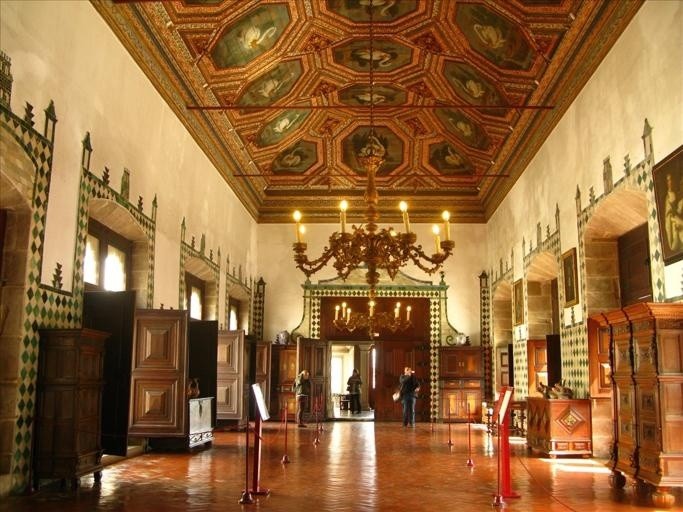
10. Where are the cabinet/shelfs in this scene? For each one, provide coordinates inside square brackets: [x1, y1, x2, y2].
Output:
[602, 300, 681, 498]
[520, 396, 593, 458]
[440, 347, 484, 423]
[149, 392, 214, 451]
[268, 344, 302, 422]
[28, 324, 112, 490]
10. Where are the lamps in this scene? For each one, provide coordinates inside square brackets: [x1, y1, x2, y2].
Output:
[332, 290, 413, 343]
[292, 2, 456, 298]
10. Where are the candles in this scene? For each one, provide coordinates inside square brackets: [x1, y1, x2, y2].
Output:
[441, 210, 452, 241]
[368, 300, 376, 317]
[346, 307, 352, 320]
[339, 199, 349, 235]
[334, 305, 340, 320]
[341, 302, 347, 319]
[292, 208, 303, 243]
[406, 305, 412, 321]
[394, 302, 401, 319]
[400, 200, 412, 235]
[432, 224, 443, 253]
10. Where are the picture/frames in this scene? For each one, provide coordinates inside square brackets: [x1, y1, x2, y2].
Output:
[510, 277, 525, 327]
[558, 247, 580, 310]
[651, 145, 683, 267]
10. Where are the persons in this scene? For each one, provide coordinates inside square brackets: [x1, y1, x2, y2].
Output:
[400, 366, 421, 429]
[663, 173, 683, 250]
[295, 368, 311, 428]
[347, 368, 362, 414]
